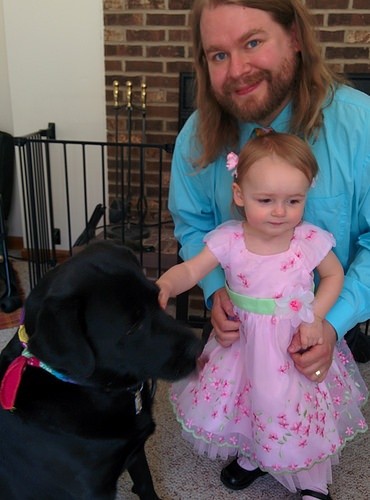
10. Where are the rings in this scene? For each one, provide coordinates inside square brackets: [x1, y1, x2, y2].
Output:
[315, 370, 323, 379]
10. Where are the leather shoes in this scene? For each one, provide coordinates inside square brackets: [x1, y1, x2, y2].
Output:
[217, 449, 272, 492]
[299, 490, 337, 500]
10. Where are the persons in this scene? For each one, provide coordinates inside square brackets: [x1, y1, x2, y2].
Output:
[167, 0, 370, 384]
[156, 128, 370, 500]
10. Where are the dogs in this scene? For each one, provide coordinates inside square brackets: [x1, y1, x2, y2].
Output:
[0, 240, 206, 500]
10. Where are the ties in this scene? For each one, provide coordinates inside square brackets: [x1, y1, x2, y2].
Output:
[255, 128, 272, 137]
